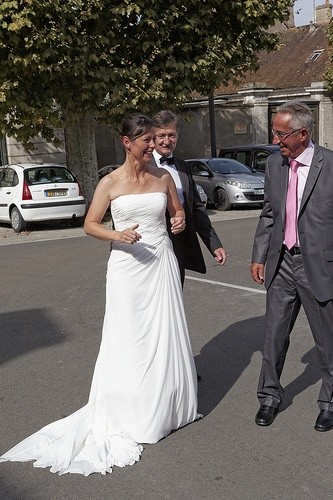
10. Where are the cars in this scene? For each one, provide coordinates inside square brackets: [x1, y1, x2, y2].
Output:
[184, 157, 266, 211]
[0, 160, 88, 233]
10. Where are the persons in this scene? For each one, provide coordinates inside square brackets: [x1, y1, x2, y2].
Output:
[83, 111, 204, 444]
[110, 110, 227, 382]
[250, 100, 333, 431]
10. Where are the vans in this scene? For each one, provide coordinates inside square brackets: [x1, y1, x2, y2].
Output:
[217, 144, 281, 174]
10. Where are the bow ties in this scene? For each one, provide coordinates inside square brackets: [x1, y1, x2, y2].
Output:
[159, 156, 176, 166]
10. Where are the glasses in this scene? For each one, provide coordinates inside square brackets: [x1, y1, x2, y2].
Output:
[271, 127, 307, 138]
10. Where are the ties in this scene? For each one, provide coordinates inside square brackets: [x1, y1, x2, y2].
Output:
[284, 160, 302, 250]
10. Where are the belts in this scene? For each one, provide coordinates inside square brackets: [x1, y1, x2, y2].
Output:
[282, 244, 302, 256]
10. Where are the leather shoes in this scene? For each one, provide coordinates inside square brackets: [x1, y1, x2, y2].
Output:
[314, 409, 333, 432]
[254, 405, 279, 426]
